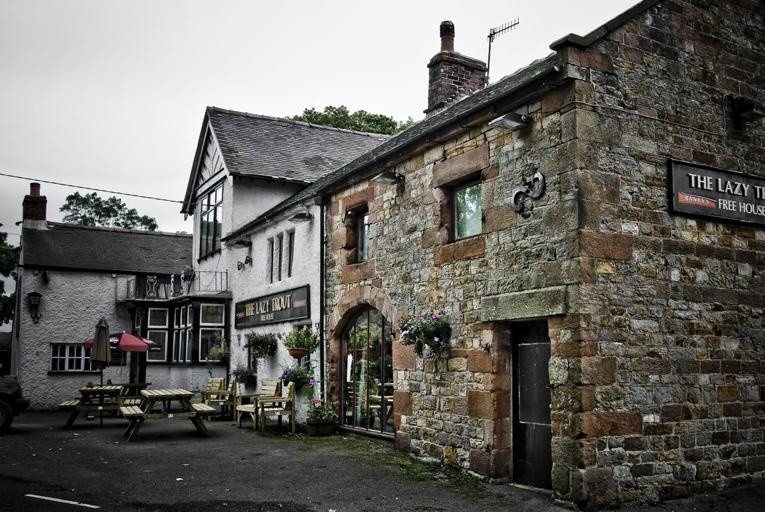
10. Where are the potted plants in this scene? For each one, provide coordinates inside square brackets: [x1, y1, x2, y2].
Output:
[276, 326, 321, 357]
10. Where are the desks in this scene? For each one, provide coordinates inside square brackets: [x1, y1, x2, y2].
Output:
[63, 385, 124, 431]
[121, 388, 212, 443]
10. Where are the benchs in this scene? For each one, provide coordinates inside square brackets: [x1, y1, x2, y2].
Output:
[191, 402, 216, 414]
[119, 405, 145, 417]
[123, 398, 141, 405]
[58, 399, 81, 406]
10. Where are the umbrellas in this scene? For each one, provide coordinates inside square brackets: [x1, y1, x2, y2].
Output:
[91, 315, 115, 423]
[84, 330, 161, 384]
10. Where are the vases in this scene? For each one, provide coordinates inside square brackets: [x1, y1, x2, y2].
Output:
[306, 418, 336, 437]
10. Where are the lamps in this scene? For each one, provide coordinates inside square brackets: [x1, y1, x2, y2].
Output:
[26, 291, 42, 324]
[728, 93, 765, 130]
[371, 171, 405, 187]
[488, 111, 534, 131]
[288, 212, 314, 222]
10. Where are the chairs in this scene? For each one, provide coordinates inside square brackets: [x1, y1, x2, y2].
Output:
[201, 378, 296, 437]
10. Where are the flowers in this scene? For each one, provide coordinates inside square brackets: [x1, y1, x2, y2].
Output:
[306, 398, 336, 420]
